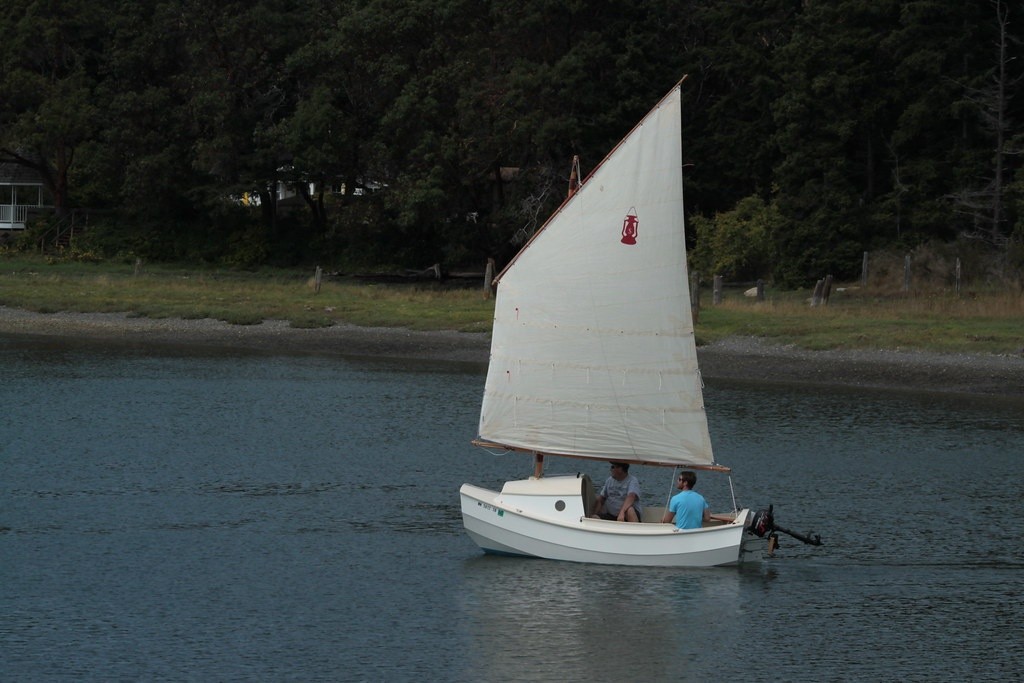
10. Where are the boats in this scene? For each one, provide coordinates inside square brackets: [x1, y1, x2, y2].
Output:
[459, 71, 823, 567]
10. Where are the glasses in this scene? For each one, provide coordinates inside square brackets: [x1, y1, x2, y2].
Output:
[611, 465, 617, 469]
[678, 477, 682, 481]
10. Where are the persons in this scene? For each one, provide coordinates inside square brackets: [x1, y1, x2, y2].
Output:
[589, 457, 645, 523]
[660, 470, 712, 532]
